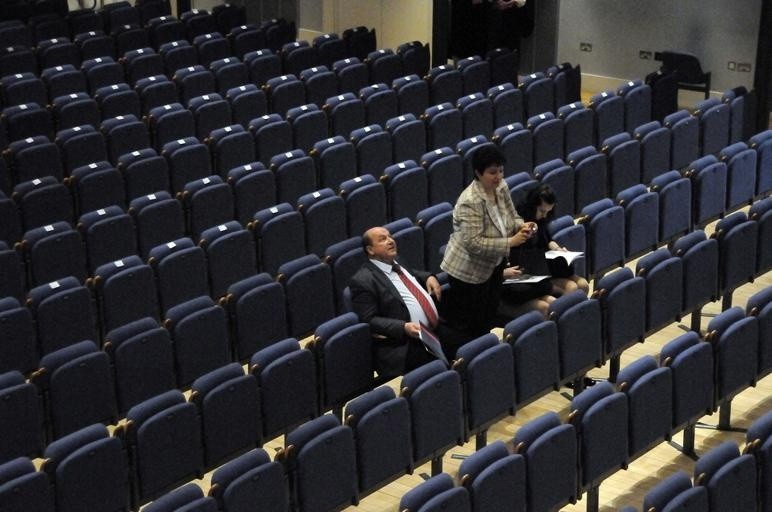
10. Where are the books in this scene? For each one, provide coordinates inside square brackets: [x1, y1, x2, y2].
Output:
[416, 319, 450, 367]
[544, 246, 585, 266]
[503, 272, 553, 286]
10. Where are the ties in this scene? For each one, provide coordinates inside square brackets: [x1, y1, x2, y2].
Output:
[393, 263, 438, 328]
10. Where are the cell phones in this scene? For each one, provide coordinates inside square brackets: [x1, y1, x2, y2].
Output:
[528, 223, 535, 229]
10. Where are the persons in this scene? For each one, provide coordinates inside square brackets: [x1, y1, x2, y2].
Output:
[438, 143, 538, 340]
[349, 226, 482, 372]
[512, 183, 590, 296]
[491, 253, 556, 319]
